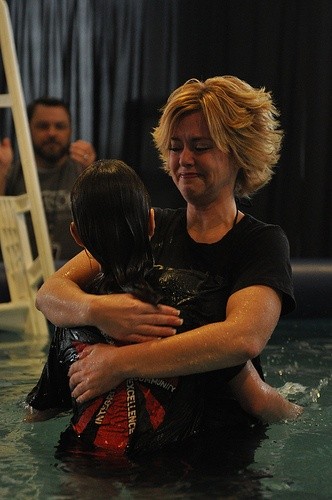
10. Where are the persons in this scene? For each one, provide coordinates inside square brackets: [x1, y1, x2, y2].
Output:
[0, 97, 96, 306]
[26, 159, 304, 500]
[34, 75, 298, 499]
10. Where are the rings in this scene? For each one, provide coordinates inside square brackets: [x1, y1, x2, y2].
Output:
[83, 153, 89, 160]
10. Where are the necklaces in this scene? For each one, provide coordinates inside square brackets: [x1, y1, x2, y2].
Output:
[233, 206, 239, 227]
